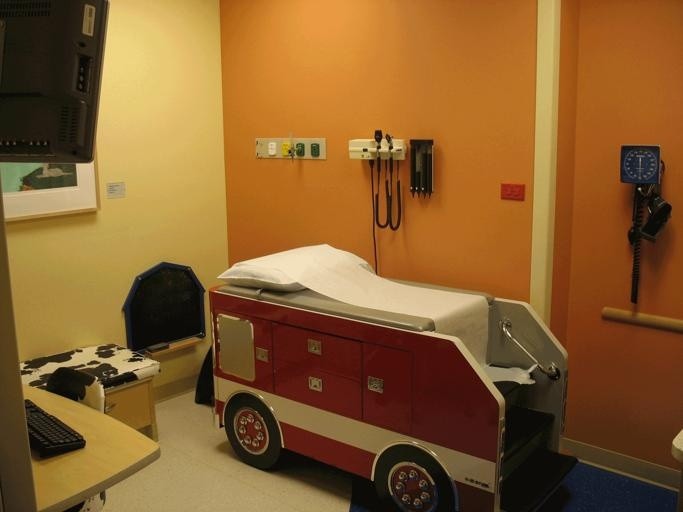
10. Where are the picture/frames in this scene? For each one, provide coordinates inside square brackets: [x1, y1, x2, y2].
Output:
[0, 133, 102, 223]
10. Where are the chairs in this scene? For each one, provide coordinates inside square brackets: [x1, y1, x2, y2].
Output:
[45, 367, 105, 512]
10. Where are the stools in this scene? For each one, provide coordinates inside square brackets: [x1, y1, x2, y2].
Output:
[19, 342, 161, 443]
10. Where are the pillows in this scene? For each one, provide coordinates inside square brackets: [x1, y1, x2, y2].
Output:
[217, 245, 378, 294]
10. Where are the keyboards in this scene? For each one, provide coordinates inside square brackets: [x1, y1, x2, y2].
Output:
[23, 398, 85, 458]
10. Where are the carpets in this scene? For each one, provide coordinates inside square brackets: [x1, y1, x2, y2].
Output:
[349, 460, 682, 511]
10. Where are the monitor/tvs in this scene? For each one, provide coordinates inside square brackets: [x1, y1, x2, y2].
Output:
[0, 0, 110, 163]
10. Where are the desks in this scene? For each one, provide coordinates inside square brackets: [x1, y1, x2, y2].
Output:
[22, 384, 161, 512]
